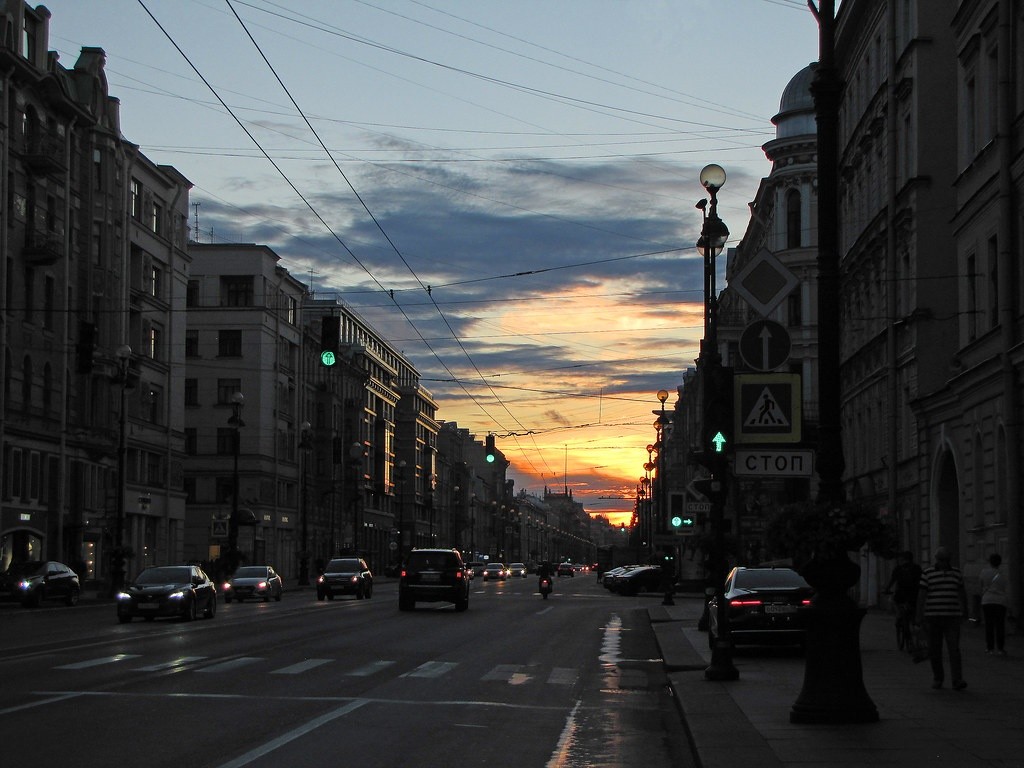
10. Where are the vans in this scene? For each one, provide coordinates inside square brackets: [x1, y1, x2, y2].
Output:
[470, 561, 486, 580]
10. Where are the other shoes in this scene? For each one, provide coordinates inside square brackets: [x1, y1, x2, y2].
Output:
[997, 649, 1008, 656]
[951, 680, 968, 689]
[985, 648, 995, 655]
[931, 679, 943, 689]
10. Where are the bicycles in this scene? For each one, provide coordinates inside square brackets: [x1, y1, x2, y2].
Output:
[894, 598, 931, 664]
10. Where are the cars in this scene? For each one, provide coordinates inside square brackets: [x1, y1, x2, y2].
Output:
[484, 563, 507, 581]
[557, 563, 576, 578]
[704, 564, 817, 656]
[506, 562, 527, 578]
[602, 564, 673, 596]
[0, 560, 82, 611]
[116, 563, 218, 622]
[223, 566, 284, 603]
[528, 562, 558, 574]
[574, 563, 598, 572]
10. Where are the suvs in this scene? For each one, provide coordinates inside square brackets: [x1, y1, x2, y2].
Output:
[316, 557, 373, 600]
[398, 547, 473, 612]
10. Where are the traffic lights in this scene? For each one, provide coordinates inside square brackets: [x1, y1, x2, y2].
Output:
[486, 436, 495, 463]
[702, 367, 731, 463]
[320, 316, 339, 367]
[670, 514, 695, 528]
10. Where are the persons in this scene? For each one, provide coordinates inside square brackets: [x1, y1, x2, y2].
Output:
[596, 563, 604, 583]
[916, 546, 967, 691]
[536, 560, 554, 593]
[886, 551, 923, 612]
[977, 554, 1011, 656]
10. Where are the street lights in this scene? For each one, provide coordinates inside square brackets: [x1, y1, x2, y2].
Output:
[470, 492, 475, 544]
[643, 444, 654, 546]
[428, 473, 436, 548]
[397, 459, 407, 578]
[694, 164, 731, 481]
[454, 486, 459, 547]
[107, 343, 132, 596]
[224, 392, 244, 571]
[349, 442, 361, 558]
[300, 420, 311, 582]
[492, 501, 551, 563]
[653, 420, 663, 519]
[656, 389, 669, 530]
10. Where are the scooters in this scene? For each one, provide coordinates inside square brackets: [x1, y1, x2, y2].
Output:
[538, 573, 554, 600]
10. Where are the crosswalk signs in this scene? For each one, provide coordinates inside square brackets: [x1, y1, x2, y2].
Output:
[734, 374, 800, 443]
